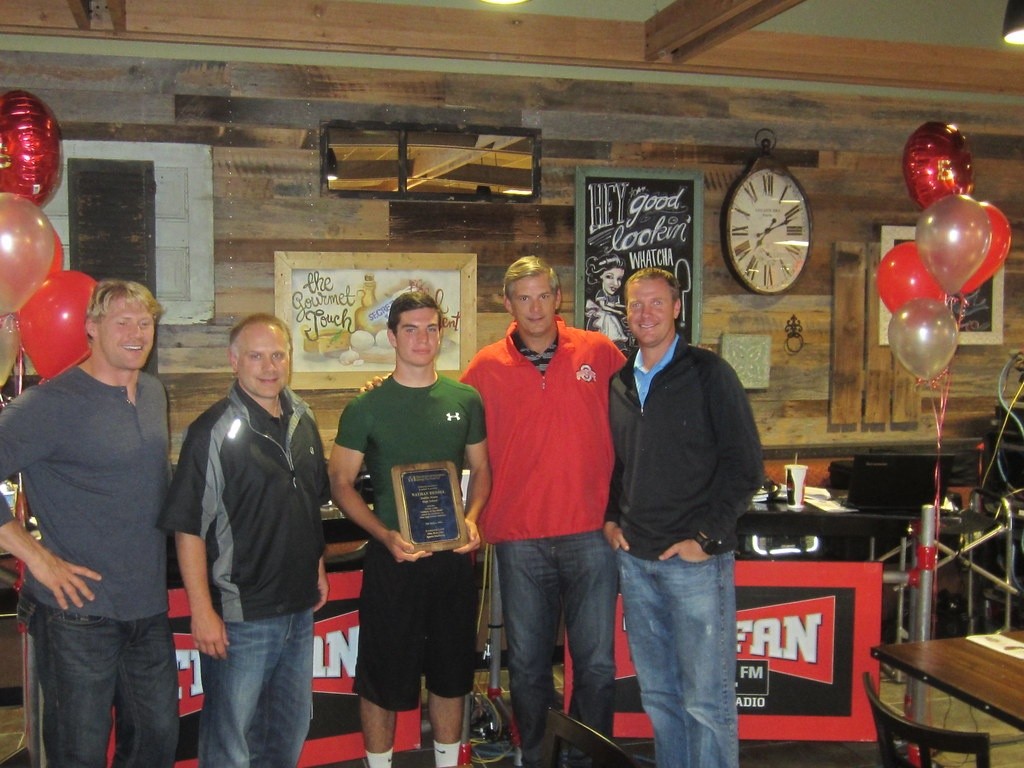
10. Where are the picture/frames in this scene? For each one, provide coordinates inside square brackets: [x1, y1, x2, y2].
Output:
[274, 250, 477, 390]
[878, 225, 1005, 347]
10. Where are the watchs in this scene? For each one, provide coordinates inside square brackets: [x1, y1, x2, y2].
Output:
[693, 533, 720, 555]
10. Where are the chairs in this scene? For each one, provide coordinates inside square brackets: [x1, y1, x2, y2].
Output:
[862, 670, 990, 768]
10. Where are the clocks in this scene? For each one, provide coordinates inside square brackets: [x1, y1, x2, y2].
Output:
[719, 137, 814, 297]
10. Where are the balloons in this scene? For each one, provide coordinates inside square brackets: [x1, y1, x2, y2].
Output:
[0, 89, 97, 388]
[876, 242, 946, 314]
[887, 299, 958, 381]
[903, 121, 973, 211]
[915, 195, 1012, 299]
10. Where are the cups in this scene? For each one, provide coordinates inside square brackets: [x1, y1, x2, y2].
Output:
[784, 464, 809, 508]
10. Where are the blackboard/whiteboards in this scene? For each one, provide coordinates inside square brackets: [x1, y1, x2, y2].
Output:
[575, 166, 702, 350]
[894, 238, 993, 334]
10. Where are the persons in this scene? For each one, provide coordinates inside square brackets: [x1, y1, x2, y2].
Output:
[360, 257, 631, 768]
[604, 267, 765, 768]
[327, 291, 490, 768]
[0, 280, 180, 768]
[157, 314, 333, 768]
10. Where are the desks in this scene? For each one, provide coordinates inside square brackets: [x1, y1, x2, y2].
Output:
[734, 487, 997, 562]
[870, 630, 1024, 733]
